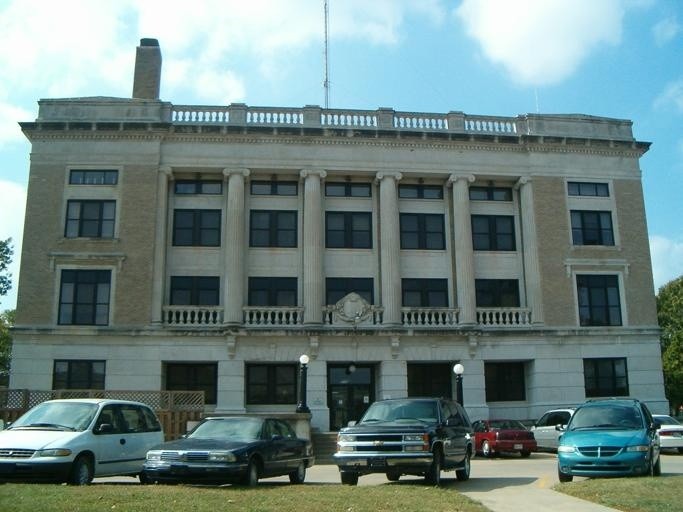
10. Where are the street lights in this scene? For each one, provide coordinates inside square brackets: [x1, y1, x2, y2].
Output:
[295, 355, 312, 413]
[452, 363, 465, 406]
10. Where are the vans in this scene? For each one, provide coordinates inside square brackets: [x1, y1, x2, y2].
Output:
[529, 408, 577, 449]
[0, 398, 166, 485]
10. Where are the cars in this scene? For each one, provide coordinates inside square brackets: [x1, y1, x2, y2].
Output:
[141, 417, 315, 486]
[471, 420, 537, 457]
[638, 415, 683, 453]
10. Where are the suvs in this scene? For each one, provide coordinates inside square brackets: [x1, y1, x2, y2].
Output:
[332, 397, 476, 485]
[555, 398, 661, 482]
